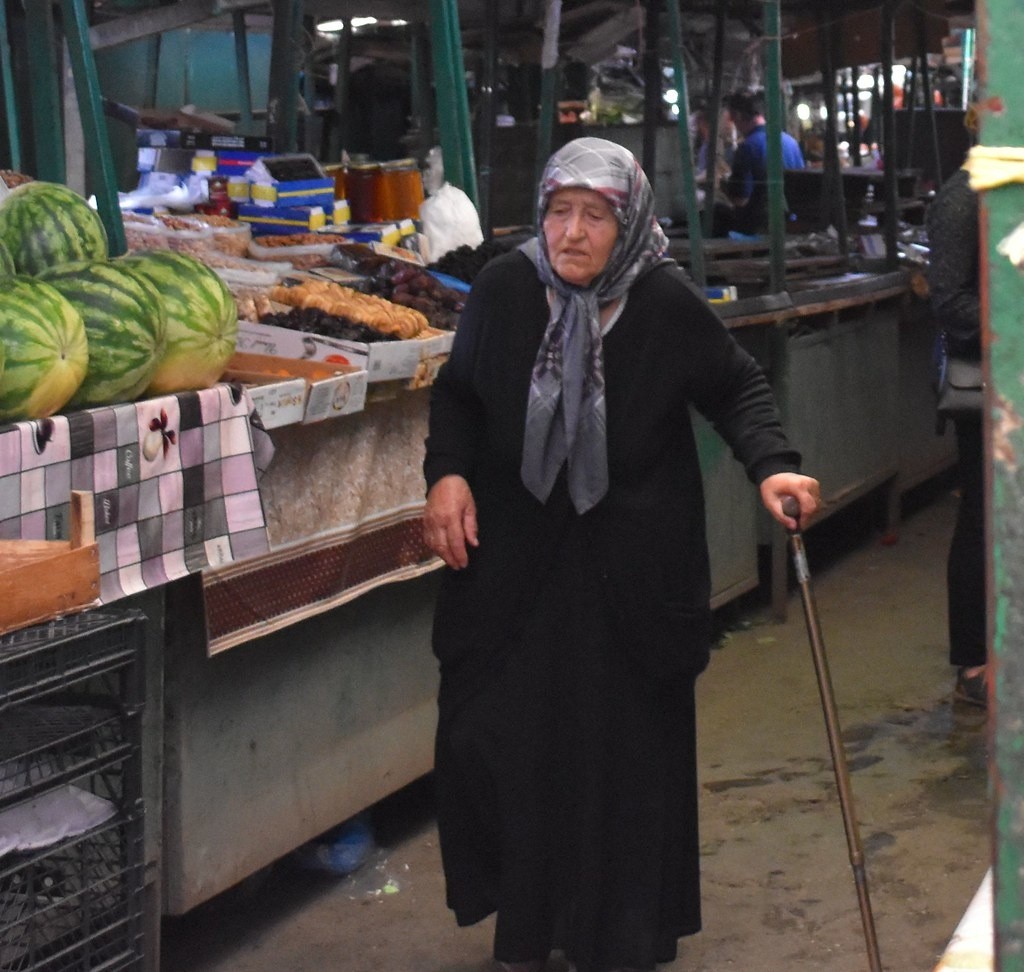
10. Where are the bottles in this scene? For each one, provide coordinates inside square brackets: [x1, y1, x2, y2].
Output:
[318, 156, 426, 225]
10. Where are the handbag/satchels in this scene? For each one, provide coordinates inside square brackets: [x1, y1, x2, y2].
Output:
[935, 359, 985, 412]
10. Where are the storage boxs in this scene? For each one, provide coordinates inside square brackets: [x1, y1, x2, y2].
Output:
[0, 605, 152, 972]
[131, 128, 470, 431]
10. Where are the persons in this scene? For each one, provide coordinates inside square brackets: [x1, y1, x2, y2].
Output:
[419, 136, 821, 972]
[910, 102, 992, 706]
[715, 90, 803, 232]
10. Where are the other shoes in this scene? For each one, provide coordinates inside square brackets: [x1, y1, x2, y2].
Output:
[954, 669, 990, 707]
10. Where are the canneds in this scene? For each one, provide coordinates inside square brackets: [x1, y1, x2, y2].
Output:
[319, 158, 425, 224]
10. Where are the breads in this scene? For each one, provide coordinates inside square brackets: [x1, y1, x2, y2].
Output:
[271, 279, 434, 339]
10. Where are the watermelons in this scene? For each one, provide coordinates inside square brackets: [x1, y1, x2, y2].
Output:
[0, 235, 16, 284]
[0, 273, 89, 423]
[0, 180, 109, 276]
[33, 259, 169, 408]
[111, 249, 238, 397]
